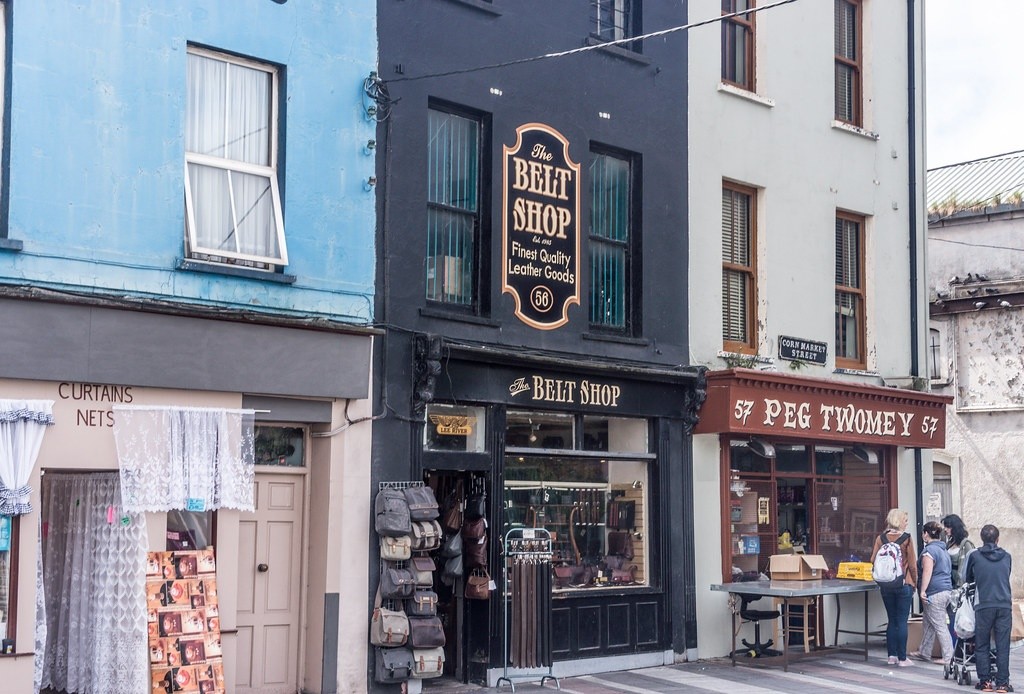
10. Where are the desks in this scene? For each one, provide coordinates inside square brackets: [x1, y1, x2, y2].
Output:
[710, 577, 880, 671]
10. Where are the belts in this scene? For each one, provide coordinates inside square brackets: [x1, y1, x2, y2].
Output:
[510, 540, 553, 668]
[573, 489, 600, 527]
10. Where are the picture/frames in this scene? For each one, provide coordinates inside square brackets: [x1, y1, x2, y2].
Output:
[848, 508, 879, 555]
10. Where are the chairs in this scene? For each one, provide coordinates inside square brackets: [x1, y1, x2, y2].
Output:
[772, 594, 821, 654]
[730, 574, 783, 659]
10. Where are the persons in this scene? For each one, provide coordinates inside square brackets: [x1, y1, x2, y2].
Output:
[871, 508, 918, 667]
[920, 514, 1013, 692]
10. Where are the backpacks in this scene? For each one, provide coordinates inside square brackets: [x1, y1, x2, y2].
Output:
[375, 483, 412, 560]
[872, 532, 911, 588]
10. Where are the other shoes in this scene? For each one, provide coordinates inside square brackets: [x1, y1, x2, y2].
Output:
[909, 651, 931, 661]
[934, 658, 950, 665]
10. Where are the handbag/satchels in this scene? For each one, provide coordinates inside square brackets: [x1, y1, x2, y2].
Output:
[436, 481, 491, 600]
[405, 486, 446, 679]
[606, 496, 635, 559]
[953, 597, 976, 639]
[554, 556, 634, 586]
[370, 607, 414, 683]
[989, 601, 1024, 652]
[380, 568, 415, 600]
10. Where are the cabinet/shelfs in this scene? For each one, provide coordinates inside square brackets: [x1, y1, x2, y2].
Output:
[730, 473, 883, 573]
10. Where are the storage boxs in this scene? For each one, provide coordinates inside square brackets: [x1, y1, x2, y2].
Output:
[732, 536, 760, 556]
[836, 562, 873, 581]
[768, 554, 829, 581]
[877, 612, 942, 658]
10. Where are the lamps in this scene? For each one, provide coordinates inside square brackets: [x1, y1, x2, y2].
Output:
[747, 435, 776, 459]
[851, 442, 879, 464]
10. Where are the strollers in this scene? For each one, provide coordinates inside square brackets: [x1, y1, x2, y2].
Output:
[943, 582, 1009, 686]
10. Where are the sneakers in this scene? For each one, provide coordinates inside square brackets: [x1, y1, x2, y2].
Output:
[888, 655, 899, 665]
[975, 679, 993, 691]
[996, 682, 1014, 692]
[898, 657, 914, 667]
